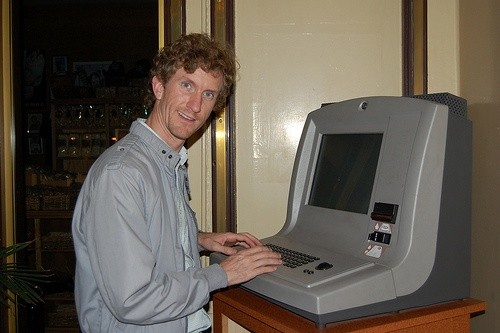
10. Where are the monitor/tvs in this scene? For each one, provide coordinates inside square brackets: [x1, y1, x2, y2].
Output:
[306, 134, 384, 215]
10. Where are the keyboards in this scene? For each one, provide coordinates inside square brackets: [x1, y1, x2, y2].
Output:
[264, 243, 318, 270]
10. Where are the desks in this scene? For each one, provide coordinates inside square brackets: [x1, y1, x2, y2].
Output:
[209, 285, 488, 333]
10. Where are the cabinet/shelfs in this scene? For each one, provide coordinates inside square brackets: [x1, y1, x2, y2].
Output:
[51, 96, 147, 177]
[18, 211, 81, 332]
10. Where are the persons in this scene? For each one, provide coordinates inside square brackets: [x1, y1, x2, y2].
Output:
[70, 33, 284, 333]
